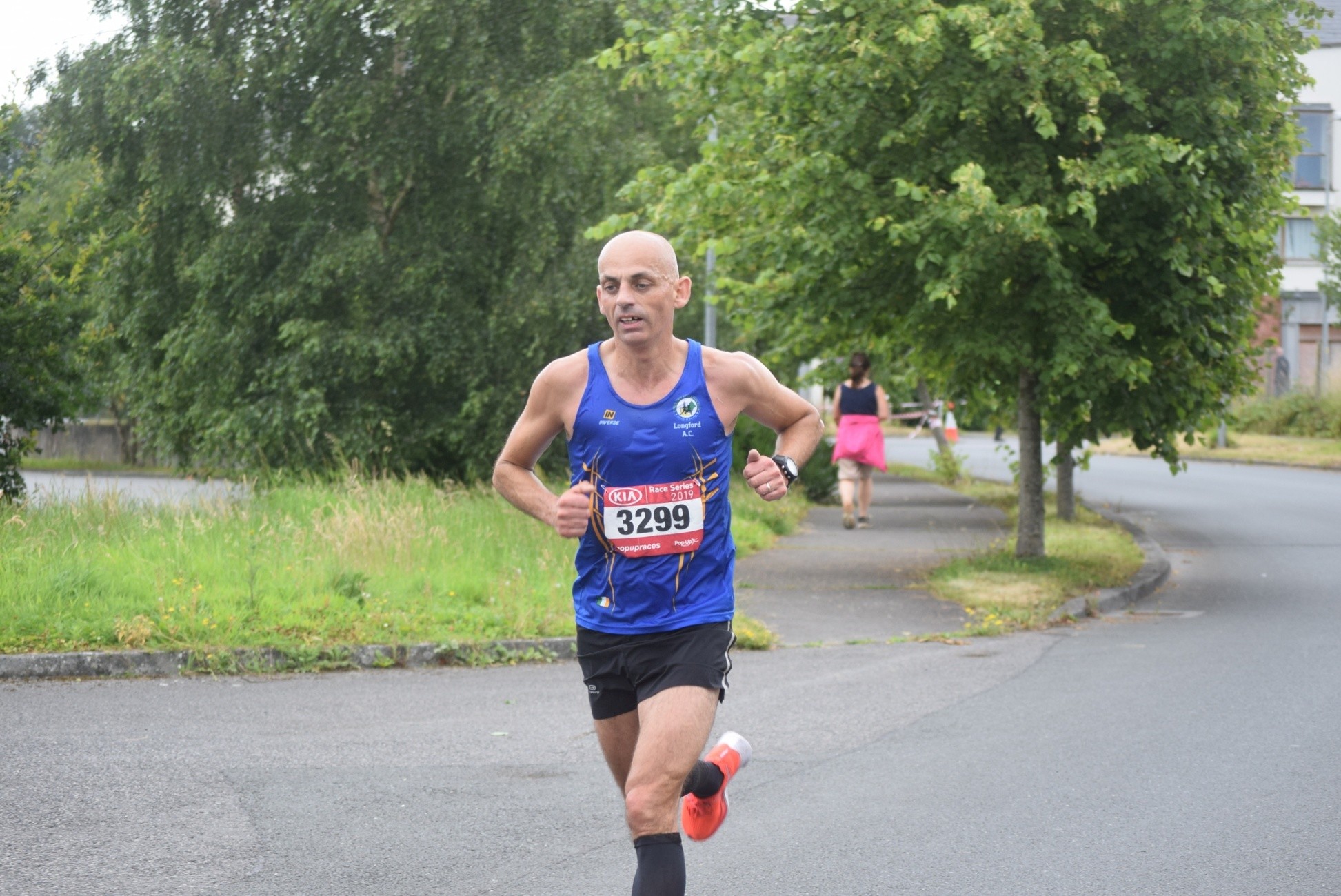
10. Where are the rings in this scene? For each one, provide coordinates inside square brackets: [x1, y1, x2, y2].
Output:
[766, 482, 772, 493]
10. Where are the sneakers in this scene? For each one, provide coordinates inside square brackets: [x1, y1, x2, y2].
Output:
[680, 731, 752, 842]
[841, 513, 854, 530]
[858, 516, 871, 525]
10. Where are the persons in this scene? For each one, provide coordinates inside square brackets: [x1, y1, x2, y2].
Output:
[831, 351, 890, 530]
[944, 402, 959, 450]
[491, 229, 825, 896]
[995, 425, 1004, 442]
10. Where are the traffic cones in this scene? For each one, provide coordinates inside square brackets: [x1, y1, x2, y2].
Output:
[943, 405, 961, 445]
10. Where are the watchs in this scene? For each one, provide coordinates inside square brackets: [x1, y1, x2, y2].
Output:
[772, 454, 799, 490]
